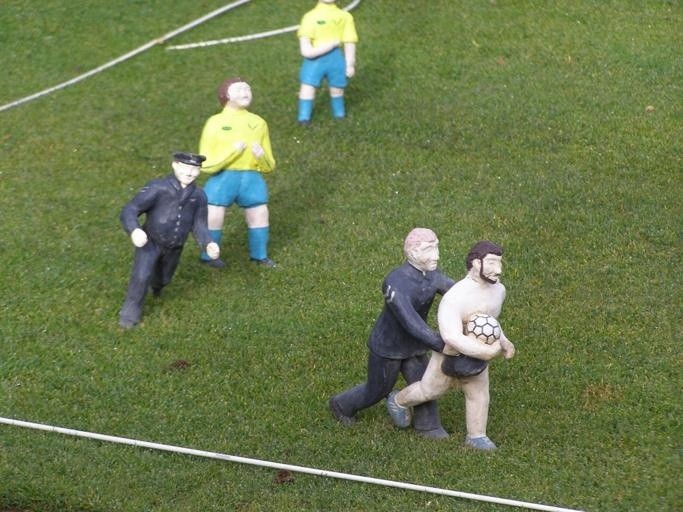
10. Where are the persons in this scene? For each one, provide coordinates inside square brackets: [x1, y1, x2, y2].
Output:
[328, 225, 461, 440]
[384, 240, 516, 453]
[116, 151, 222, 329]
[193, 75, 278, 270]
[295, 0, 361, 126]
